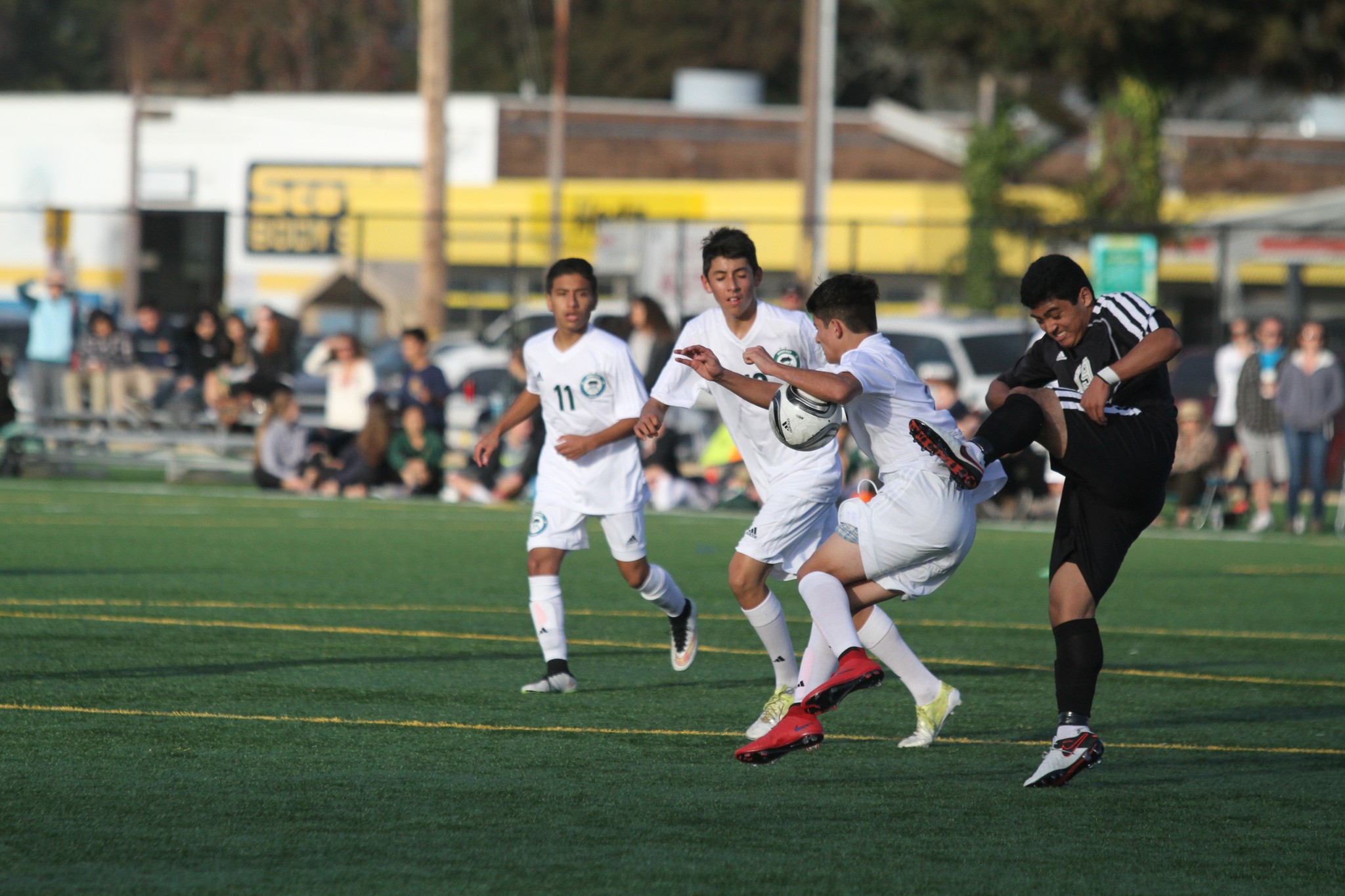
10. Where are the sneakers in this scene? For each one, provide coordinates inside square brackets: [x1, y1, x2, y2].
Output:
[909, 419, 985, 490]
[668, 597, 697, 671]
[898, 681, 961, 747]
[746, 684, 794, 741]
[522, 672, 578, 694]
[1025, 724, 1104, 787]
[735, 702, 823, 765]
[801, 647, 884, 715]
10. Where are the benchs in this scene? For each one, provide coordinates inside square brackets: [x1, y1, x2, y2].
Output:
[15, 403, 327, 484]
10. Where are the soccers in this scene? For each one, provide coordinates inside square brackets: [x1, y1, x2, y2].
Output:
[769, 382, 842, 451]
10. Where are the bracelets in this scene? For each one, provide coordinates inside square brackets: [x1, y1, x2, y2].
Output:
[1095, 367, 1121, 387]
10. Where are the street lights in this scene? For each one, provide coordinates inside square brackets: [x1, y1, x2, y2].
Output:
[123, 108, 169, 317]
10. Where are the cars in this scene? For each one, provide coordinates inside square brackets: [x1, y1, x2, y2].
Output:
[878, 314, 1045, 412]
[438, 293, 635, 387]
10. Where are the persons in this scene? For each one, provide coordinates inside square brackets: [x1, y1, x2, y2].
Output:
[301, 333, 379, 479]
[618, 297, 1063, 516]
[1, 271, 282, 484]
[661, 271, 1009, 763]
[634, 227, 965, 750]
[320, 392, 392, 498]
[1149, 397, 1219, 531]
[1273, 321, 1345, 533]
[375, 403, 442, 500]
[441, 339, 543, 504]
[380, 327, 448, 436]
[471, 257, 699, 694]
[1235, 317, 1293, 532]
[908, 251, 1181, 790]
[1215, 320, 1255, 531]
[253, 391, 320, 493]
